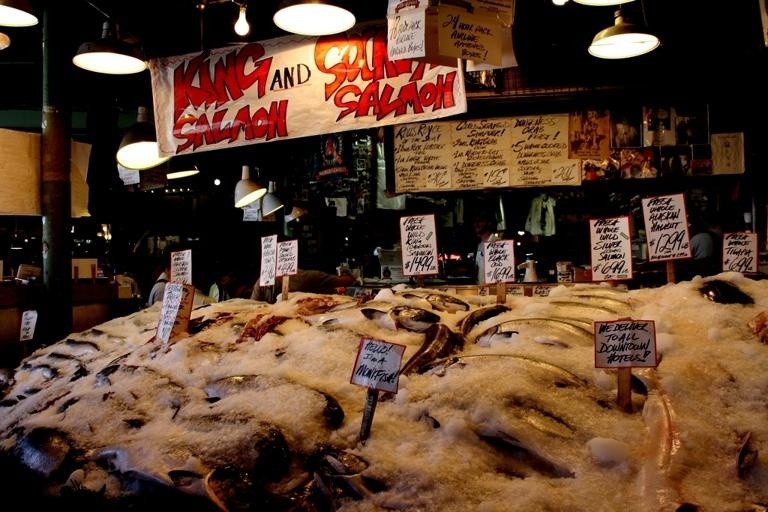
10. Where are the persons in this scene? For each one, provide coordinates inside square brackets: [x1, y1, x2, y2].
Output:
[685, 217, 713, 280]
[471, 208, 500, 284]
[208, 261, 247, 303]
[146, 242, 189, 306]
[249, 234, 356, 302]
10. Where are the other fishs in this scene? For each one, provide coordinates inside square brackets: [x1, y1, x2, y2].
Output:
[0, 280, 768, 511]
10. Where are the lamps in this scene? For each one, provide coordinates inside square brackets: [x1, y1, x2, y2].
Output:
[72, 0, 152, 73]
[234, 160, 314, 222]
[126, 169, 195, 196]
[165, 155, 201, 182]
[115, 103, 173, 170]
[554, 0, 673, 62]
[269, 2, 361, 38]
[1, 1, 42, 29]
